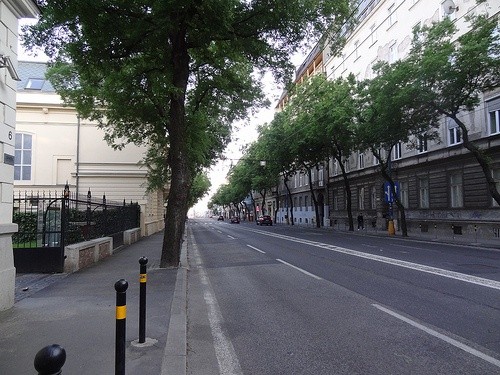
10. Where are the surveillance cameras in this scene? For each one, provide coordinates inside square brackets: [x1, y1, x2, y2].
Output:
[0, 54, 21, 81]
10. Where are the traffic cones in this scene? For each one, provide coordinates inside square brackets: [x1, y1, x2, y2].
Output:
[388, 221, 396, 235]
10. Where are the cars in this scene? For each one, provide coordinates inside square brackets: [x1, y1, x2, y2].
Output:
[256, 215, 273, 226]
[229, 215, 240, 224]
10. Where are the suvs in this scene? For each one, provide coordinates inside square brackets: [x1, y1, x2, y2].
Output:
[217, 215, 224, 221]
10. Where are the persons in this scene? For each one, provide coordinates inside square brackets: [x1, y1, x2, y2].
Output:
[357, 212, 366, 231]
